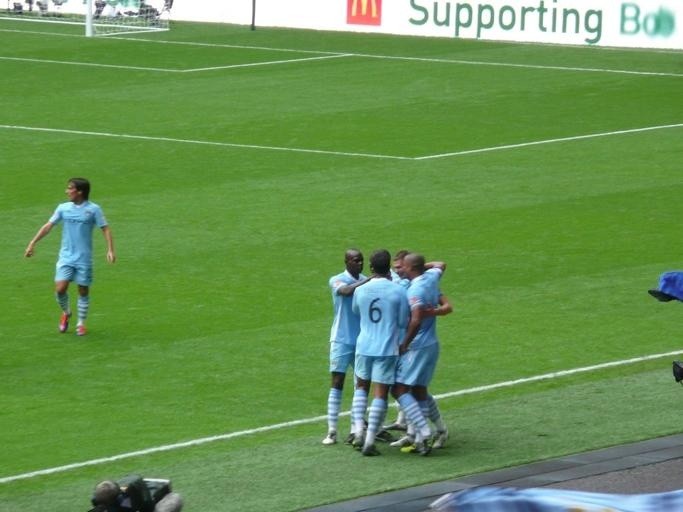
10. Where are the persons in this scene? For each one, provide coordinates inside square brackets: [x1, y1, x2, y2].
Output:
[24, 178, 116, 334]
[90, 479, 126, 512]
[321, 244, 451, 456]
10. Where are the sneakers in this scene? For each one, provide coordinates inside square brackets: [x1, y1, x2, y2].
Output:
[343, 416, 380, 456]
[375, 430, 395, 442]
[381, 422, 407, 430]
[321, 431, 335, 445]
[59, 310, 72, 333]
[390, 428, 447, 456]
[75, 323, 86, 336]
